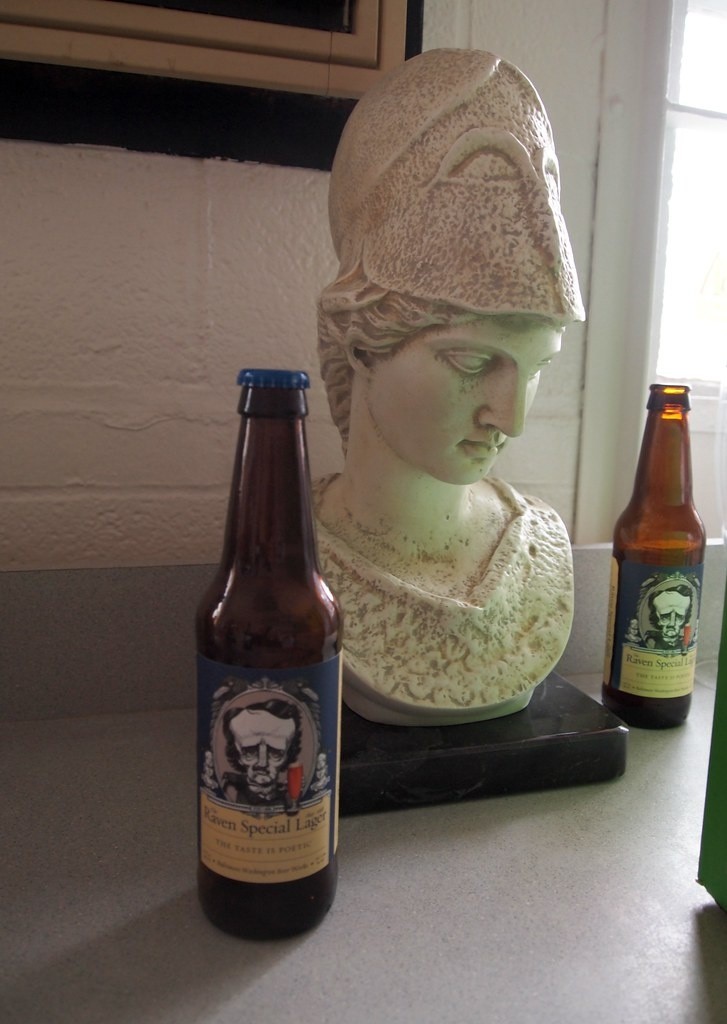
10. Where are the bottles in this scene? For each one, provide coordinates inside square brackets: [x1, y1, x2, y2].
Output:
[193, 369, 343, 937]
[601, 383, 706, 729]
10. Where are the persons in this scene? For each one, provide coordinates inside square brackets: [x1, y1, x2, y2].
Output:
[315, 46, 589, 728]
